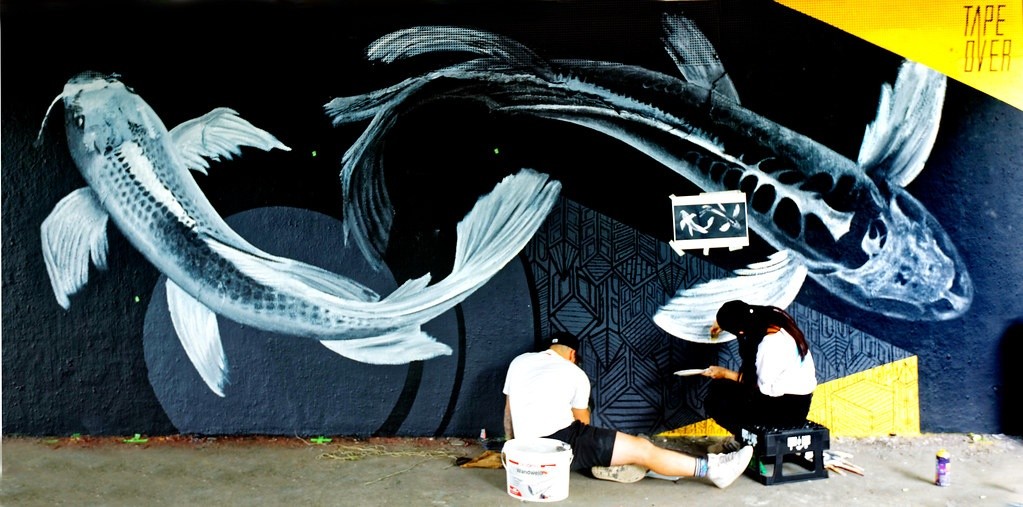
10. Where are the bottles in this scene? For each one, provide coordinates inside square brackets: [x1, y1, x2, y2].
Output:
[478, 428, 488, 451]
[935, 446, 950, 487]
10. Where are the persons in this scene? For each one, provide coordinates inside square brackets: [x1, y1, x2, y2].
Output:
[703, 299, 818, 454]
[502, 331, 753, 491]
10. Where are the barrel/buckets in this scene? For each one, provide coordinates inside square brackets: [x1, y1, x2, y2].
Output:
[501, 438, 573, 502]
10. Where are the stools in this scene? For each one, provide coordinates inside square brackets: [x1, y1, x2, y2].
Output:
[735, 421, 830, 485]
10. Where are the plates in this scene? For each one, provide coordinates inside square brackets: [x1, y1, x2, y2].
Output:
[673, 369, 710, 376]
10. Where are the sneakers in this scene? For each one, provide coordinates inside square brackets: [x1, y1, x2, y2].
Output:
[591, 464, 647, 483]
[705, 445, 753, 489]
[707, 436, 740, 454]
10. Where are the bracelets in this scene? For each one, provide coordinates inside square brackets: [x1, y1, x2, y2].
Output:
[736, 371, 741, 384]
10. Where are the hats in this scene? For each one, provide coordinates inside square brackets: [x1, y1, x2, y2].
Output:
[717, 300, 757, 339]
[547, 331, 584, 364]
